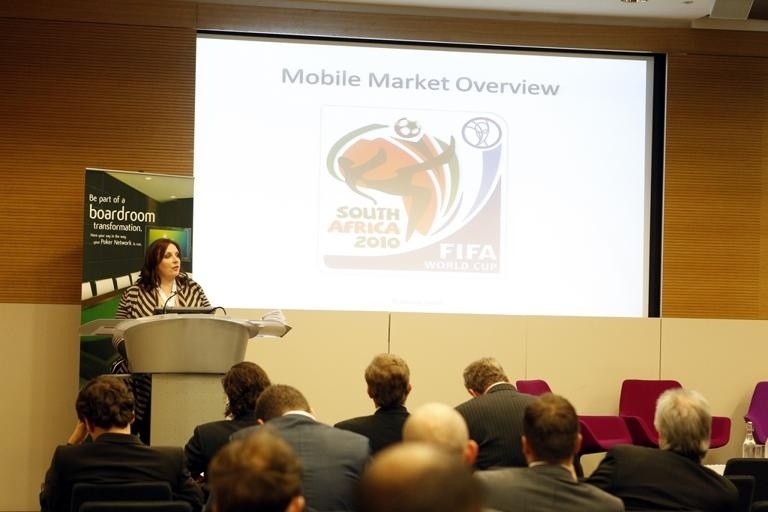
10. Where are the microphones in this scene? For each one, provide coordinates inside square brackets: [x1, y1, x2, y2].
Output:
[163, 286, 186, 314]
[208, 306, 226, 316]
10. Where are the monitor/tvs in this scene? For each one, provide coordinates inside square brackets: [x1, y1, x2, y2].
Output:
[154, 306, 217, 315]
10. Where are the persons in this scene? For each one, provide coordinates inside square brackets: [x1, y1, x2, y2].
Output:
[588, 387, 742, 511]
[35, 375, 205, 512]
[361, 441, 496, 512]
[184, 361, 271, 476]
[207, 432, 308, 512]
[229, 384, 370, 512]
[451, 356, 538, 467]
[472, 391, 626, 512]
[333, 352, 412, 456]
[400, 402, 479, 465]
[110, 238, 212, 445]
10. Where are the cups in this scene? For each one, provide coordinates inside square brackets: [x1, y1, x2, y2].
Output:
[753, 443, 767, 459]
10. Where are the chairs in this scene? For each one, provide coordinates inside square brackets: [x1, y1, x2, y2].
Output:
[723, 475, 757, 512]
[78, 501, 194, 512]
[516, 379, 634, 480]
[744, 382, 768, 457]
[70, 481, 174, 512]
[724, 458, 768, 501]
[618, 379, 731, 449]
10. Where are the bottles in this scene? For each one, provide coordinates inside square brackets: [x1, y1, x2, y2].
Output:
[740, 420, 755, 459]
